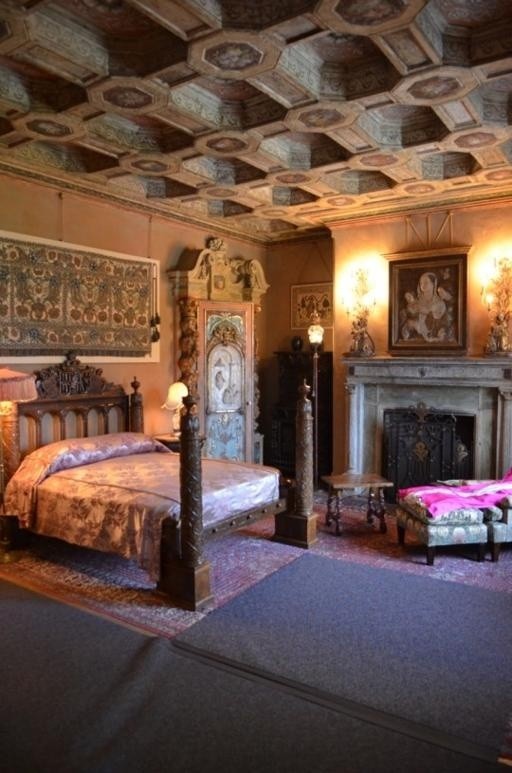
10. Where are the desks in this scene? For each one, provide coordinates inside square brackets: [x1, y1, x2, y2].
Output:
[322, 474, 393, 535]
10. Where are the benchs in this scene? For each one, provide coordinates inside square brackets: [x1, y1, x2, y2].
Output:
[396, 479, 512, 562]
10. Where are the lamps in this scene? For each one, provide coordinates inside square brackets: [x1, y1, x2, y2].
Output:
[307, 312, 324, 352]
[161, 382, 191, 438]
[0, 367, 38, 483]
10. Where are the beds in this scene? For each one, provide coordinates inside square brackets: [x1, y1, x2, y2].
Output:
[0, 353, 295, 597]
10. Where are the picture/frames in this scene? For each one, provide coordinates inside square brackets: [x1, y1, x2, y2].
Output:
[290, 281, 333, 330]
[386, 253, 469, 355]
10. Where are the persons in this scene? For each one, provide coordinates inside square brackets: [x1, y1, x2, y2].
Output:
[400, 270, 455, 342]
